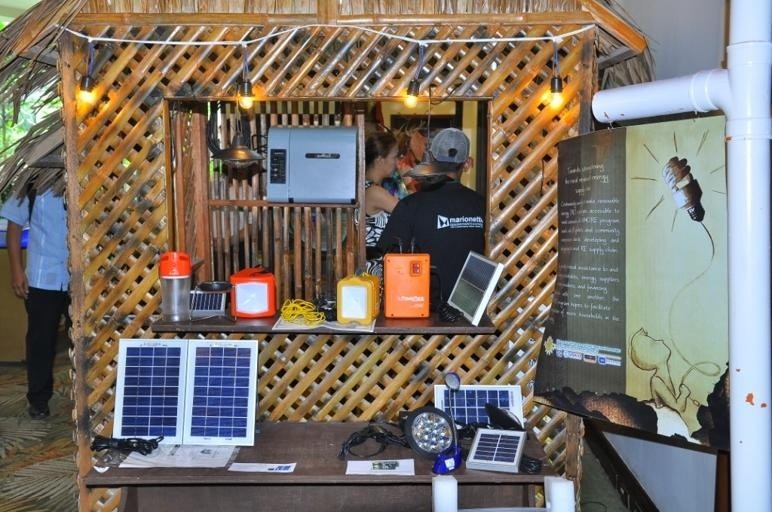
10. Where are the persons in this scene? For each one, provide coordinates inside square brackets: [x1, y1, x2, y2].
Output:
[354, 133, 403, 306]
[374, 128, 487, 309]
[1, 185, 69, 418]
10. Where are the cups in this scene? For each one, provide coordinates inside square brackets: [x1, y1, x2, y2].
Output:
[159, 252, 191, 322]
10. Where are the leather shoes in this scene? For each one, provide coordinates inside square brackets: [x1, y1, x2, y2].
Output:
[29, 400, 51, 420]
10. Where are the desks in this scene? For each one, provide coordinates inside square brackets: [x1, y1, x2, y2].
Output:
[83, 420, 564, 512]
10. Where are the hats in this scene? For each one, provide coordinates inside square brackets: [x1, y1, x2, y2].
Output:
[430, 126, 470, 165]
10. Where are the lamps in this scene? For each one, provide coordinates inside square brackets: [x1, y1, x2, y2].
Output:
[238, 80, 256, 110]
[210, 128, 264, 168]
[404, 79, 420, 108]
[76, 71, 95, 106]
[546, 76, 563, 106]
